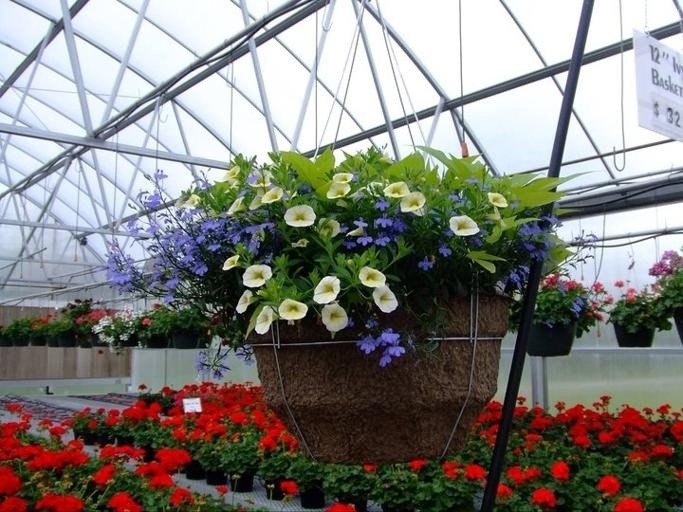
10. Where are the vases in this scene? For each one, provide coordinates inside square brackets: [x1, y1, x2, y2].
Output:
[523, 321, 574, 358]
[250, 292, 512, 468]
